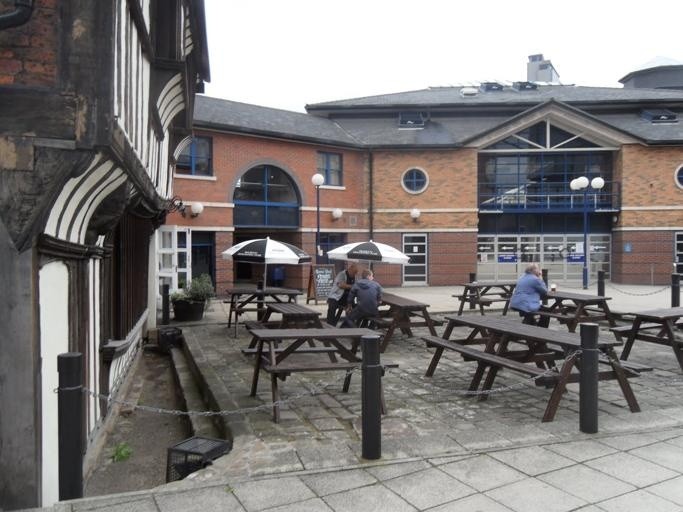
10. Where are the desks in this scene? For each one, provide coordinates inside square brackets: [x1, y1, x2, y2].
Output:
[620, 306, 683, 371]
[451, 282, 618, 329]
[425, 316, 642, 420]
[249, 327, 391, 423]
[378, 292, 439, 353]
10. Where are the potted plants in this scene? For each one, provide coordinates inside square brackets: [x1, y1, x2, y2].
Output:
[170, 273, 215, 321]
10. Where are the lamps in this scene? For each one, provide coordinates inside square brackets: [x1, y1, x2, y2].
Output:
[411, 209, 421, 222]
[191, 201, 204, 218]
[332, 207, 342, 222]
[167, 195, 186, 219]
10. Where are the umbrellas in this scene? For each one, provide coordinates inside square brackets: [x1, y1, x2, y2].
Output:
[326, 240, 410, 271]
[220, 235, 312, 307]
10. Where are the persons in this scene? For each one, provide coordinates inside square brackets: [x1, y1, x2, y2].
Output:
[326, 264, 359, 327]
[510, 263, 548, 326]
[344, 268, 384, 328]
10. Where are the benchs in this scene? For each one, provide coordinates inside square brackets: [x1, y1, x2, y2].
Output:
[521, 337, 651, 372]
[422, 335, 559, 382]
[413, 310, 445, 322]
[341, 303, 385, 327]
[609, 317, 683, 332]
[263, 362, 363, 373]
[221, 287, 341, 348]
[241, 348, 351, 354]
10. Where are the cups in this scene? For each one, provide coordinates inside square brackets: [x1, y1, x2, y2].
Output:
[551, 284, 557, 296]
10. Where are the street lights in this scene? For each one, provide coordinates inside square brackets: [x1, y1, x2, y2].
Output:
[569, 174, 605, 290]
[311, 174, 324, 263]
